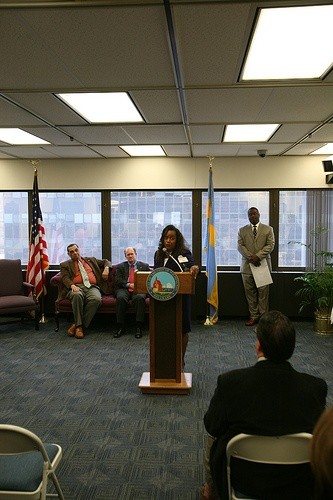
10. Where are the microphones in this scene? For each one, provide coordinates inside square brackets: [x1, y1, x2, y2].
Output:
[163, 248, 183, 272]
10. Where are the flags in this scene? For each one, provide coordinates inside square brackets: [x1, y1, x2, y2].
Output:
[204, 172, 218, 324]
[26, 176, 49, 302]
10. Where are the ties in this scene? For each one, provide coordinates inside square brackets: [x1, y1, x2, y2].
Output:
[128, 264, 134, 292]
[77, 259, 91, 288]
[253, 226, 257, 239]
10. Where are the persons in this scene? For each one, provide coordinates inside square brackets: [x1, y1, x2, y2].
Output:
[113, 247, 149, 338]
[154, 225, 199, 371]
[60, 244, 112, 339]
[237, 207, 275, 326]
[201, 310, 328, 500]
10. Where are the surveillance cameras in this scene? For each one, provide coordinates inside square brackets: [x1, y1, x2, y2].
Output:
[257, 150, 267, 158]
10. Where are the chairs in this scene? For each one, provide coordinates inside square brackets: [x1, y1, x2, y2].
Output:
[0, 260, 40, 331]
[226, 432, 313, 500]
[0, 424, 65, 500]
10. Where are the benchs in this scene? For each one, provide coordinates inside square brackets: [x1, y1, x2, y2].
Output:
[50, 265, 154, 332]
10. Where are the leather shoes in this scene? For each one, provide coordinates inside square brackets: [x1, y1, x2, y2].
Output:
[245, 320, 254, 326]
[113, 329, 124, 338]
[135, 328, 142, 339]
[67, 324, 75, 337]
[76, 327, 84, 339]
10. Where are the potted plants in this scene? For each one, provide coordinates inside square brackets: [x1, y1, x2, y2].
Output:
[288, 226, 333, 336]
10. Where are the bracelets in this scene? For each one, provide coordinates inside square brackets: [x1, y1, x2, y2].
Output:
[105, 266, 109, 268]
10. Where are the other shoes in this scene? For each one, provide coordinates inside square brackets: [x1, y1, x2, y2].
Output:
[202, 480, 210, 497]
